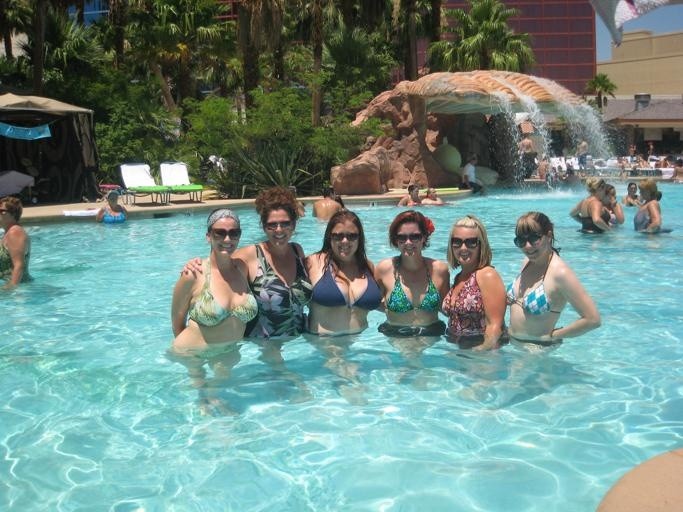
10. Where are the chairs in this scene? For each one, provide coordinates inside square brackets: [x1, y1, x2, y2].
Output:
[160, 162, 204, 204]
[120, 164, 171, 208]
[547, 155, 675, 175]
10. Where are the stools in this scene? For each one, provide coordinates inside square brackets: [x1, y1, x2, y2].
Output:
[100, 184, 119, 206]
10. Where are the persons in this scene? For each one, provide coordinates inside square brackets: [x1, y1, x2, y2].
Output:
[441, 214, 510, 352]
[170, 207, 258, 382]
[394, 183, 444, 207]
[596, 447, 683, 512]
[504, 211, 601, 353]
[208, 155, 229, 199]
[0, 197, 35, 292]
[96, 189, 129, 224]
[372, 210, 452, 336]
[286, 183, 350, 223]
[180, 187, 314, 347]
[302, 210, 387, 338]
[460, 128, 682, 237]
